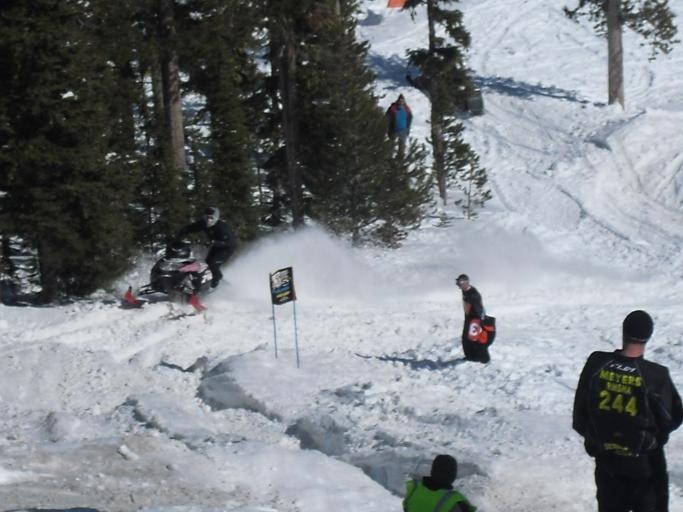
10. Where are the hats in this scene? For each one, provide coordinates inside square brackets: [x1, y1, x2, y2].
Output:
[204, 206, 219, 217]
[431, 454, 456, 484]
[623, 311, 653, 342]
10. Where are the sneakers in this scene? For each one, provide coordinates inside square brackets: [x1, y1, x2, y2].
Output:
[211, 271, 221, 287]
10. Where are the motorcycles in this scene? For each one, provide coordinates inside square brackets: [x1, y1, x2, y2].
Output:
[117, 239, 228, 317]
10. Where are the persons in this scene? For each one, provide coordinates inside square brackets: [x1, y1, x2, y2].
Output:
[383, 92, 415, 162]
[396, 451, 477, 511]
[570, 307, 683, 510]
[172, 205, 237, 288]
[455, 272, 493, 365]
